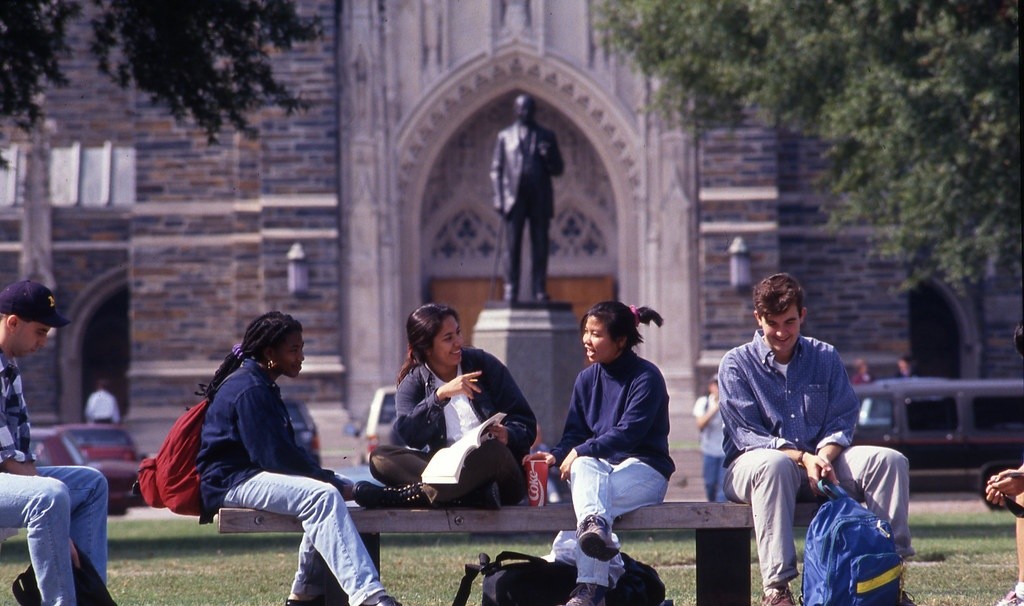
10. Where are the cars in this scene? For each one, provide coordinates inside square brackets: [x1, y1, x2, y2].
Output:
[26, 424, 143, 517]
[840, 354, 911, 386]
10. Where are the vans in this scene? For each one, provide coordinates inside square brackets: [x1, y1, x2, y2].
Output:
[852, 376, 1024, 512]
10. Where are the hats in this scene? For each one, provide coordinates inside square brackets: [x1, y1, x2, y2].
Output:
[709, 373, 718, 383]
[0, 279, 72, 329]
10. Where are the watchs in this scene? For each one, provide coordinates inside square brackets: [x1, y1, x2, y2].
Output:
[797, 450, 806, 468]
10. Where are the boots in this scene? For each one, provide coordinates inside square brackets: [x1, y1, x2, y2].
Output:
[451, 480, 503, 511]
[351, 480, 435, 509]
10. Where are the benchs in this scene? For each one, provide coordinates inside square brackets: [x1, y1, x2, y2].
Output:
[219, 503, 823, 606]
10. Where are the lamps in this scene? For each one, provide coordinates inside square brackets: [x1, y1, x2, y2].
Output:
[729, 237, 753, 292]
[286, 244, 310, 296]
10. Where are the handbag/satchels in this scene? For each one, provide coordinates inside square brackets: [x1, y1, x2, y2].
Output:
[11, 542, 118, 606]
[452, 550, 675, 606]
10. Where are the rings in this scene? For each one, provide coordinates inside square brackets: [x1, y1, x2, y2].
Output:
[505, 439, 506, 442]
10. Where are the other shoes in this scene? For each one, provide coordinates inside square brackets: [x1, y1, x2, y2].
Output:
[995, 590, 1024, 606]
[899, 587, 916, 606]
[362, 595, 402, 606]
[761, 588, 795, 606]
[285, 596, 327, 606]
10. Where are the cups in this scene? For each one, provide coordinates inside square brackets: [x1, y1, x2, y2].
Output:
[524, 459, 550, 507]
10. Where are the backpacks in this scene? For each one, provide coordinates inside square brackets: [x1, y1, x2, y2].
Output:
[131, 368, 248, 516]
[798, 478, 905, 606]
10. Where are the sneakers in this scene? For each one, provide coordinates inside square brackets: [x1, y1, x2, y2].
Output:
[575, 514, 620, 561]
[565, 583, 607, 606]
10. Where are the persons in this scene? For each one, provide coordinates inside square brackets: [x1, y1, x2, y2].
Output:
[352, 303, 538, 510]
[0, 279, 109, 606]
[84, 377, 121, 424]
[895, 357, 919, 378]
[852, 357, 876, 384]
[692, 372, 728, 503]
[521, 300, 676, 606]
[985, 320, 1024, 606]
[717, 272, 919, 606]
[194, 311, 404, 606]
[489, 93, 565, 301]
